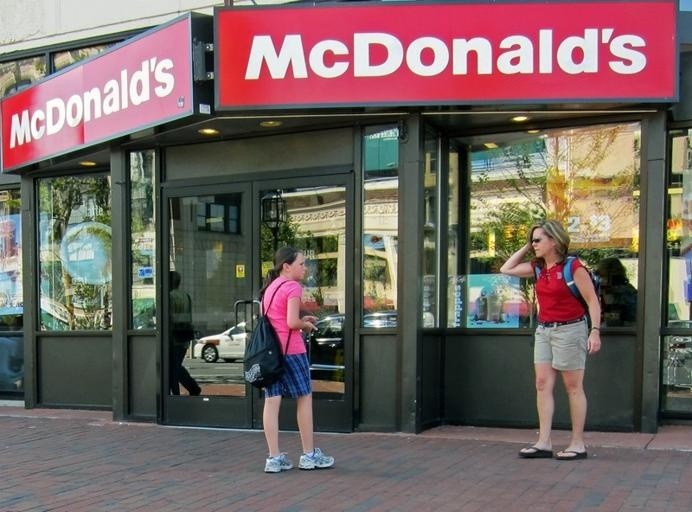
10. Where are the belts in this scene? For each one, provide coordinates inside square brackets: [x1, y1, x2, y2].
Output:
[538, 317, 586, 328]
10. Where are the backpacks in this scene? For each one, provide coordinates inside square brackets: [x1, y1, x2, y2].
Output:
[243, 314, 285, 388]
[536, 256, 602, 315]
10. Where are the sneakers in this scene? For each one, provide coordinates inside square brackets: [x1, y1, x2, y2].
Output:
[298, 448, 335, 469]
[264, 453, 294, 472]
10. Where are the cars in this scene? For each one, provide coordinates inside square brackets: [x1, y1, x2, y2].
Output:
[300, 310, 397, 381]
[192, 316, 261, 364]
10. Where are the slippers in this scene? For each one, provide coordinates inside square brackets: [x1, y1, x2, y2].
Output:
[556, 449, 587, 460]
[518, 445, 553, 458]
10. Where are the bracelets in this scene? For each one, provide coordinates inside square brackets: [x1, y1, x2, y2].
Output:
[589, 326, 600, 333]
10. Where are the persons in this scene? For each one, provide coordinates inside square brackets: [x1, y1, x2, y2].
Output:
[257, 245, 335, 474]
[592, 256, 639, 325]
[167, 270, 203, 397]
[497, 218, 605, 462]
